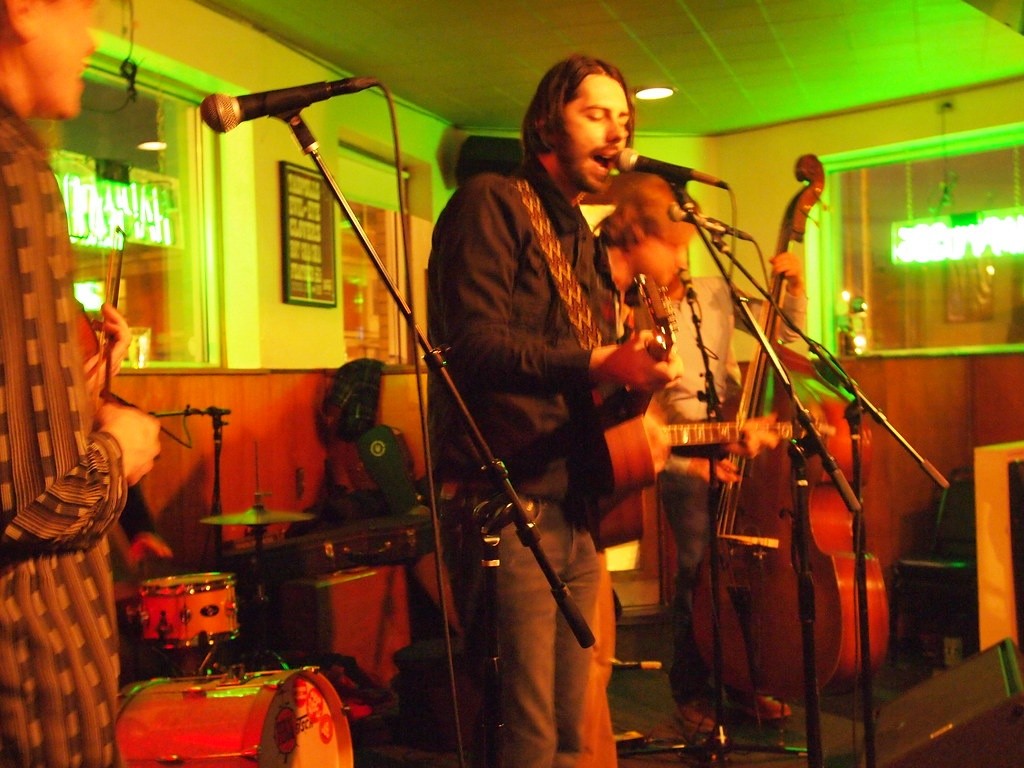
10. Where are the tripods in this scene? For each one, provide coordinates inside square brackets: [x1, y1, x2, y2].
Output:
[616, 299, 811, 768]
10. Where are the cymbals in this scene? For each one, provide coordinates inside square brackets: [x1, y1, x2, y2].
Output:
[195, 503, 318, 527]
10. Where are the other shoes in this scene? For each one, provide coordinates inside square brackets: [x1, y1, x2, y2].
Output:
[673, 696, 725, 733]
[727, 694, 791, 719]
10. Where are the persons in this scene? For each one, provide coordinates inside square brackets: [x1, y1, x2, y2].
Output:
[591, 168, 791, 735]
[116, 480, 178, 683]
[653, 245, 810, 740]
[0, 4, 159, 767]
[428, 55, 684, 767]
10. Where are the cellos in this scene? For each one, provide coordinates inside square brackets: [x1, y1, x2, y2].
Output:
[691, 152, 891, 748]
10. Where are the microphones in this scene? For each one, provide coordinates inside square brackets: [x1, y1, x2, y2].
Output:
[99, 390, 138, 410]
[200, 76, 379, 133]
[678, 268, 696, 303]
[616, 148, 731, 190]
[669, 201, 752, 240]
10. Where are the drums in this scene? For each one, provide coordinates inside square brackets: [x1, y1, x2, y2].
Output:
[116, 663, 355, 768]
[133, 565, 239, 653]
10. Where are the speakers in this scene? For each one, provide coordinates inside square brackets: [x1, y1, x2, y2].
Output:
[269, 564, 411, 725]
[862, 637, 1024, 768]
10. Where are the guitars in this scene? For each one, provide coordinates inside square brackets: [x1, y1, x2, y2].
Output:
[578, 269, 679, 555]
[658, 416, 839, 451]
